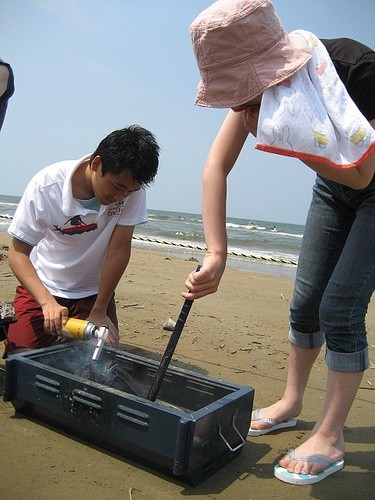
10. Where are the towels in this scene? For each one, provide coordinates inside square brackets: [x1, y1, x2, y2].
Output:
[254, 28, 375, 169]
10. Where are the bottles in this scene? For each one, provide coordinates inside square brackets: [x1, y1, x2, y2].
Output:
[44, 316, 110, 362]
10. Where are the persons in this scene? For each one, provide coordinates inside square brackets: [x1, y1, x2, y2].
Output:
[179, 0, 375, 487]
[0, 123, 163, 369]
[0, 57, 16, 134]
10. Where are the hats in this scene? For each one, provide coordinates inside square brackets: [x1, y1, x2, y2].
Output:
[188, 0, 312, 109]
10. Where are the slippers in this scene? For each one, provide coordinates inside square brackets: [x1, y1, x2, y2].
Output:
[273, 448, 344, 485]
[246, 408, 298, 437]
